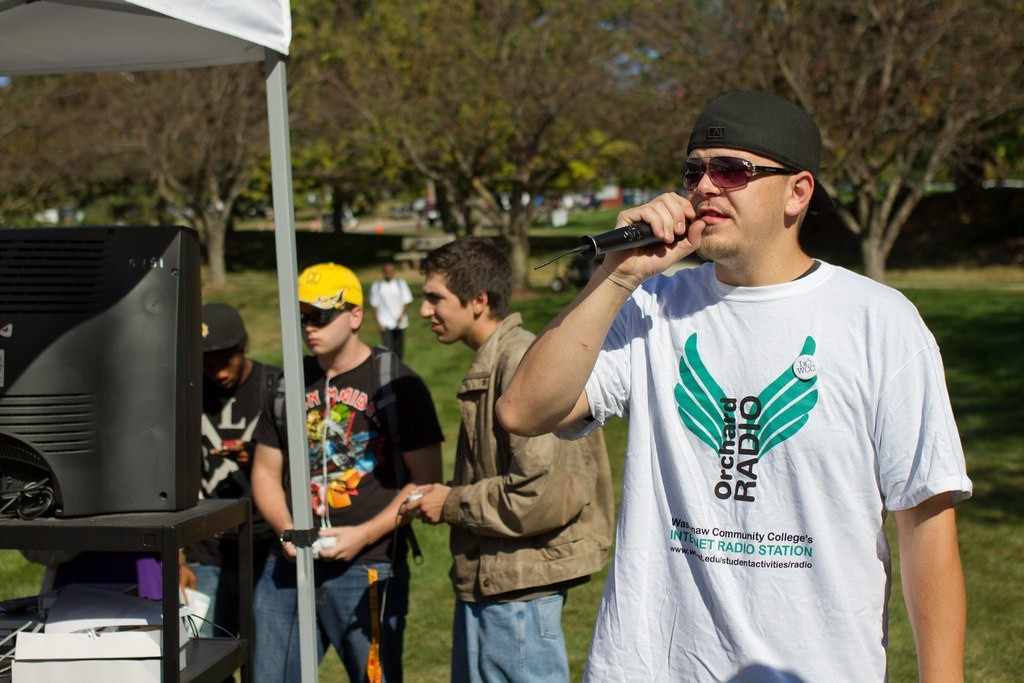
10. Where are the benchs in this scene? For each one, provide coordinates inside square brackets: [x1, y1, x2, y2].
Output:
[394, 234, 456, 271]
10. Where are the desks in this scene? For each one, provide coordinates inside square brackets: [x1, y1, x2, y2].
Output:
[0, 498, 254, 683]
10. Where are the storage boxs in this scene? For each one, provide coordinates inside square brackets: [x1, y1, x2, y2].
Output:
[12, 617, 188, 683]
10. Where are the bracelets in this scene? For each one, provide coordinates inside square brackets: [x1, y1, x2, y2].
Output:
[599, 264, 636, 292]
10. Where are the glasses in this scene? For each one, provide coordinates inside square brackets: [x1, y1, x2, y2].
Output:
[300, 305, 356, 329]
[681, 156, 799, 191]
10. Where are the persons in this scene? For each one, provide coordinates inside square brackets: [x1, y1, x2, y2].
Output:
[250, 262, 446, 683]
[398, 236, 615, 683]
[494, 88, 975, 683]
[178, 304, 284, 683]
[370, 256, 414, 360]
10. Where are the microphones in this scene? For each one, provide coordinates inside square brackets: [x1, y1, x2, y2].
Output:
[534, 217, 692, 270]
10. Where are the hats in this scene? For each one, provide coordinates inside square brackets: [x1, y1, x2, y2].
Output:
[687, 89, 834, 212]
[298, 262, 363, 309]
[202, 304, 244, 353]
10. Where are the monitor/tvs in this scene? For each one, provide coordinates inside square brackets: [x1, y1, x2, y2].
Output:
[0, 225, 202, 516]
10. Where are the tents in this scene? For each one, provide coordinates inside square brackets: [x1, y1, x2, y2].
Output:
[1, 2, 319, 683]
